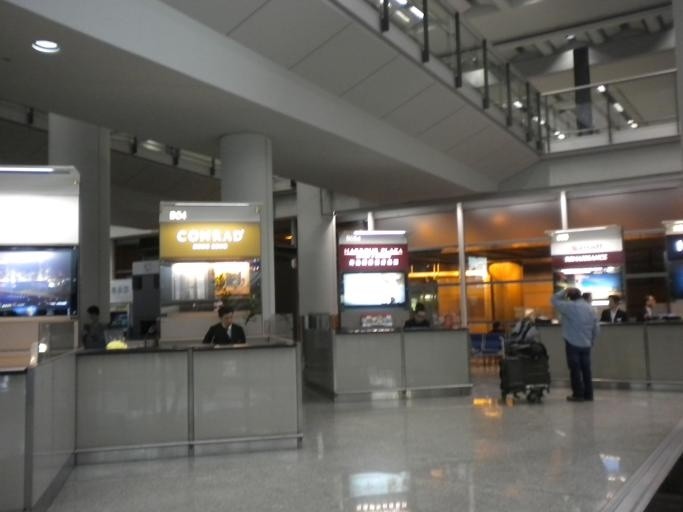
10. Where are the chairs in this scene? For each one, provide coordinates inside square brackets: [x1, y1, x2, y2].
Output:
[469, 330, 506, 366]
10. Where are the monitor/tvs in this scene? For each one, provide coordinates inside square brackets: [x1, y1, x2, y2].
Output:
[0, 243, 80, 318]
[574, 273, 625, 301]
[159, 259, 260, 306]
[340, 271, 410, 309]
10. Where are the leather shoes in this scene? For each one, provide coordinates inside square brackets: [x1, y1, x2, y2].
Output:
[566, 396, 583, 402]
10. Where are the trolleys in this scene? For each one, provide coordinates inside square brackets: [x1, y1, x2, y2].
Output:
[494, 332, 550, 402]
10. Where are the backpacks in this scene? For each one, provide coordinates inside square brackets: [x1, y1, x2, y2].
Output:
[509, 319, 537, 343]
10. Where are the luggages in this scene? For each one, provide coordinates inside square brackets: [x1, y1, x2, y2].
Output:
[500, 375, 551, 390]
[499, 359, 549, 379]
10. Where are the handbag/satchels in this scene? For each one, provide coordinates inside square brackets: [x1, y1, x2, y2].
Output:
[505, 341, 549, 361]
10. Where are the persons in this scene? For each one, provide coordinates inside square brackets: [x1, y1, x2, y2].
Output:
[600, 296, 627, 323]
[635, 293, 656, 322]
[582, 291, 594, 306]
[82, 305, 108, 349]
[201, 305, 246, 345]
[550, 286, 602, 401]
[404, 303, 431, 328]
[490, 321, 504, 334]
[433, 312, 457, 329]
